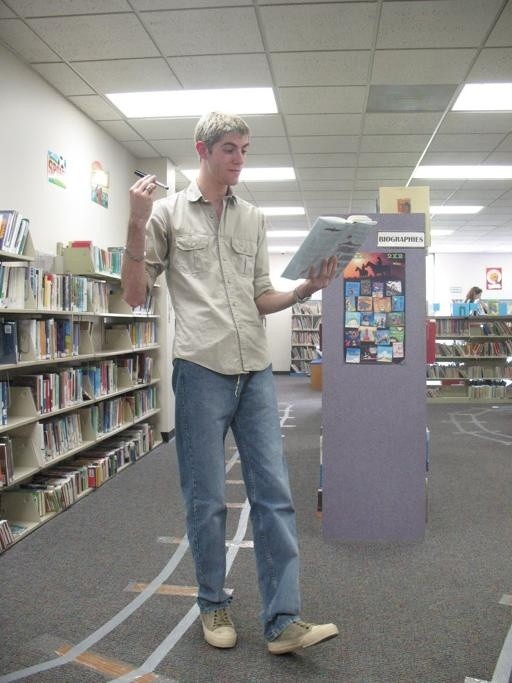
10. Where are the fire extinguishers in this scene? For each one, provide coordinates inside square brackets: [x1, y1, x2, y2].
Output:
[426, 318, 436, 363]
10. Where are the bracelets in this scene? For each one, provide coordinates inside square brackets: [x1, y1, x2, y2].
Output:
[293, 287, 312, 303]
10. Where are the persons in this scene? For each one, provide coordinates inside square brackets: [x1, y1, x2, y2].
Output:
[466, 287, 488, 315]
[361, 315, 369, 326]
[121, 111, 340, 655]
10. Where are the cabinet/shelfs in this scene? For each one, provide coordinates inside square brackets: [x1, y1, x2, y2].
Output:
[292, 300, 322, 375]
[425, 315, 512, 404]
[0, 253, 162, 555]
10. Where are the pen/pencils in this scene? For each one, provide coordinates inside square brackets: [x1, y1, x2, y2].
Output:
[135, 169, 169, 189]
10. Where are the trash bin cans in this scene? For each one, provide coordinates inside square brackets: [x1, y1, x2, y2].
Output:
[311, 359, 322, 390]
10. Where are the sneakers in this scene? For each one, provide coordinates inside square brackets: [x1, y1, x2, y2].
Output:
[267, 619, 341, 655]
[200, 606, 239, 649]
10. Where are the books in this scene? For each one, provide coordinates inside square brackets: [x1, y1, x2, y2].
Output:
[1, 352, 160, 549]
[281, 214, 378, 282]
[291, 303, 322, 374]
[425, 301, 512, 400]
[0, 209, 121, 273]
[0, 260, 158, 363]
